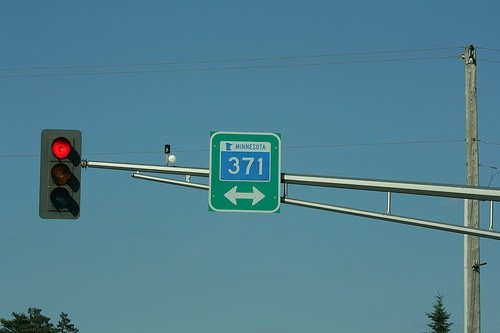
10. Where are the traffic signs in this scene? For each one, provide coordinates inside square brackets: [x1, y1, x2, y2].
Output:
[208, 130, 282, 215]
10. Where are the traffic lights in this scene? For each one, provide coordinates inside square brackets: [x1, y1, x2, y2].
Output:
[39, 129, 82, 221]
